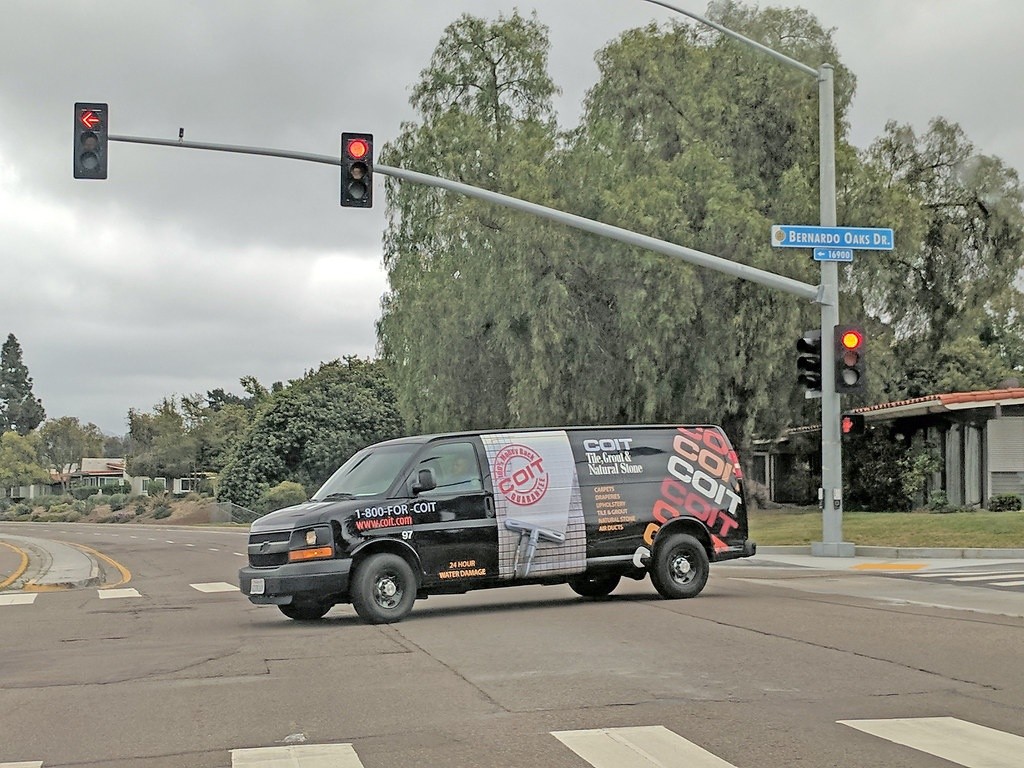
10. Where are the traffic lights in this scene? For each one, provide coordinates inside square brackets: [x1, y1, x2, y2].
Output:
[842, 413, 866, 434]
[833, 323, 869, 395]
[796, 329, 821, 398]
[340, 131, 373, 208]
[72, 100, 108, 181]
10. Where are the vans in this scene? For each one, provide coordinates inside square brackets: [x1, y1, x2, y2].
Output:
[237, 421, 759, 623]
